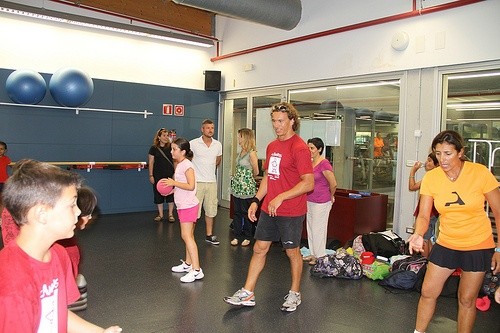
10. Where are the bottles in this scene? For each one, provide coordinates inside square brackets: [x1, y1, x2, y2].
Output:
[349, 193, 362, 199]
[359, 191, 371, 197]
[406, 160, 426, 168]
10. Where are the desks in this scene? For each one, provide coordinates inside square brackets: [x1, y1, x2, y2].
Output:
[229, 188, 388, 246]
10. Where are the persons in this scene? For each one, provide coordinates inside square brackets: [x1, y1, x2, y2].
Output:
[405, 131, 500, 333]
[0, 142, 123, 333]
[147, 128, 175, 221]
[222, 103, 315, 312]
[302, 137, 337, 265]
[161, 138, 205, 282]
[374, 133, 385, 157]
[408, 152, 440, 259]
[230, 128, 260, 246]
[188, 119, 222, 245]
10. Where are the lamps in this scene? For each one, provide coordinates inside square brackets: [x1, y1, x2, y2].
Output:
[0, 0, 215, 48]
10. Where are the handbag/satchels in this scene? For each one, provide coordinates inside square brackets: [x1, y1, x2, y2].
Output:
[362, 233, 403, 263]
[378, 269, 416, 294]
[362, 260, 389, 281]
[311, 254, 363, 280]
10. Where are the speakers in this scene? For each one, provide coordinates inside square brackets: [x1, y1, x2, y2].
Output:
[205, 70, 220, 91]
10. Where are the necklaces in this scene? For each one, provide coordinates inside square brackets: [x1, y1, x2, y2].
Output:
[445, 161, 462, 181]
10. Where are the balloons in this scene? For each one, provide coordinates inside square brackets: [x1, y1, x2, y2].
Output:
[49, 67, 95, 108]
[156, 178, 173, 195]
[5, 69, 47, 105]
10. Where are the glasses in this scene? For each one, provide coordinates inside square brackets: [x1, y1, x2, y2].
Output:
[273, 106, 287, 110]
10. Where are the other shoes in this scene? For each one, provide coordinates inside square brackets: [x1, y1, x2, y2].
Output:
[241, 239, 250, 246]
[168, 215, 175, 222]
[230, 239, 238, 245]
[154, 216, 164, 222]
[303, 255, 318, 265]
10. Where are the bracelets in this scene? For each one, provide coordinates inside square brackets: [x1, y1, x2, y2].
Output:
[149, 174, 153, 177]
[409, 176, 414, 178]
[494, 247, 500, 252]
[250, 197, 260, 208]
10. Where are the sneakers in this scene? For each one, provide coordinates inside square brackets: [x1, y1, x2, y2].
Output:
[180, 268, 204, 283]
[172, 259, 192, 273]
[280, 291, 301, 311]
[205, 235, 220, 245]
[223, 287, 255, 306]
